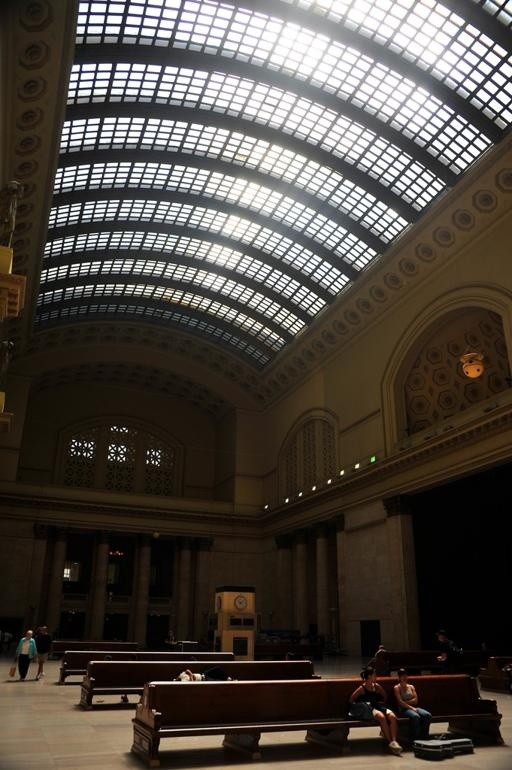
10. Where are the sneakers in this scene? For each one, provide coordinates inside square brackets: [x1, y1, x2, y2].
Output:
[391, 747, 400, 756]
[36, 671, 45, 680]
[389, 741, 402, 749]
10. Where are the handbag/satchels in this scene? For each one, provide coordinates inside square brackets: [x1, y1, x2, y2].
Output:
[346, 701, 373, 721]
[9, 663, 16, 677]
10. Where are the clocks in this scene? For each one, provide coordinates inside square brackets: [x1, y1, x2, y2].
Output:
[234, 595, 248, 611]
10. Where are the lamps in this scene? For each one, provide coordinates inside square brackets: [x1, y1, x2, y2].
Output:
[458, 328, 485, 380]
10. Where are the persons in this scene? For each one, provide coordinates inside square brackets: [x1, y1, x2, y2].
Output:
[173, 669, 233, 681]
[165, 629, 177, 645]
[14, 629, 37, 681]
[36, 626, 52, 680]
[393, 668, 433, 741]
[286, 651, 297, 660]
[350, 666, 403, 756]
[361, 644, 386, 670]
[435, 629, 459, 674]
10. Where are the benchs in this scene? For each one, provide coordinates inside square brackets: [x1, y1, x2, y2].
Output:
[373, 647, 511, 694]
[47, 638, 321, 710]
[130, 674, 506, 769]
